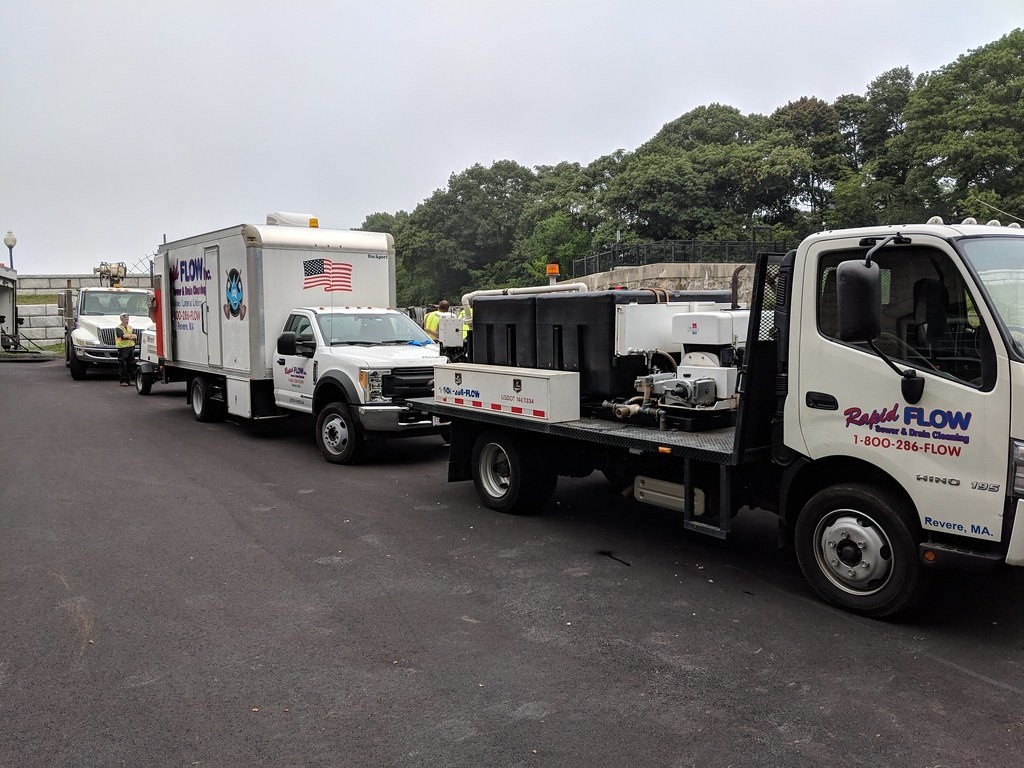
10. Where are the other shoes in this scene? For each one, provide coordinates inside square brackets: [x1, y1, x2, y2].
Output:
[129, 381, 135, 386]
[121, 382, 128, 386]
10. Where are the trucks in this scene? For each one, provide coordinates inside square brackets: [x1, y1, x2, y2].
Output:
[62, 261, 156, 381]
[0, 262, 24, 352]
[404, 214, 1024, 622]
[133, 213, 454, 465]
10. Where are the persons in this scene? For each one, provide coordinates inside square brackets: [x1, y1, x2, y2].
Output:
[422, 300, 473, 342]
[114, 312, 137, 386]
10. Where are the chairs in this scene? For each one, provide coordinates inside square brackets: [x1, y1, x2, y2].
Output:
[914, 277, 949, 348]
[85, 296, 145, 313]
[360, 318, 395, 341]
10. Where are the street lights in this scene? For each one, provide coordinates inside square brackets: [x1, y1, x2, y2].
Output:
[4, 231, 18, 270]
